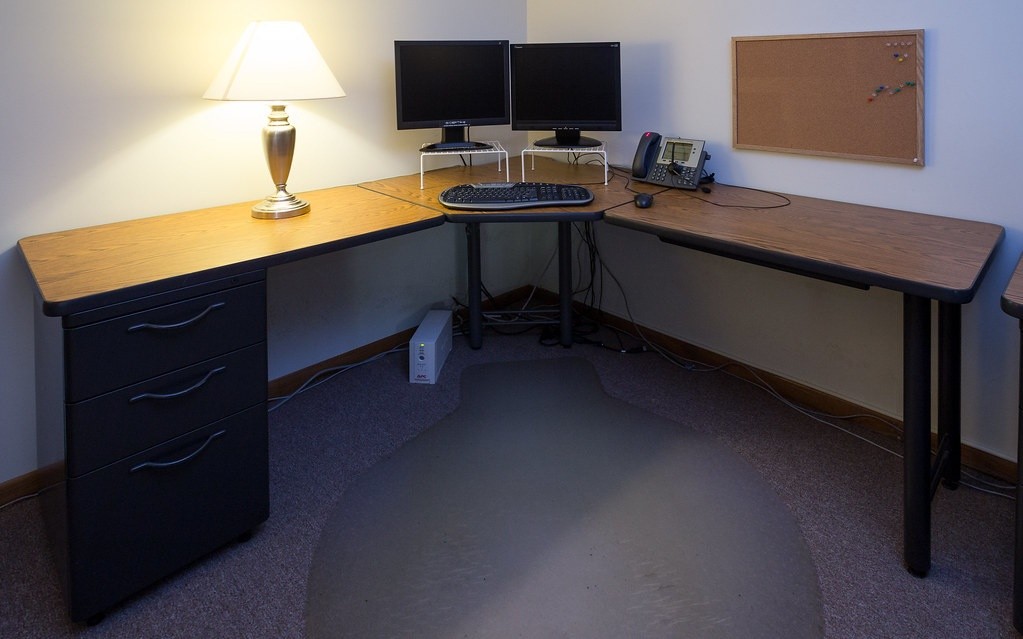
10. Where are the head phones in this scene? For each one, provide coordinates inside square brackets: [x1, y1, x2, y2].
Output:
[667, 137, 680, 175]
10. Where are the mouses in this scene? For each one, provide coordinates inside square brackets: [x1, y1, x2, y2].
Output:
[634, 193, 653, 208]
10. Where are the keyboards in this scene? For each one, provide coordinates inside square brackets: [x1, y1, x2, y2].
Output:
[438, 183, 594, 211]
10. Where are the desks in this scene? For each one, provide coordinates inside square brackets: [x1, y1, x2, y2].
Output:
[19, 156, 1007, 629]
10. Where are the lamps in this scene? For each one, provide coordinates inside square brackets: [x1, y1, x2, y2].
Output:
[203, 19, 346, 218]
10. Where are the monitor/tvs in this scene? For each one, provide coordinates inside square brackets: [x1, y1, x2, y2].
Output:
[393, 40, 509, 152]
[510, 42, 621, 147]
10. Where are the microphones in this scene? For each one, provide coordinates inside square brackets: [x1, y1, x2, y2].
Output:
[674, 170, 712, 193]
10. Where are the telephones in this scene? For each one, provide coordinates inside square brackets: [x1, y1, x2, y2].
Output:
[631, 131, 711, 191]
